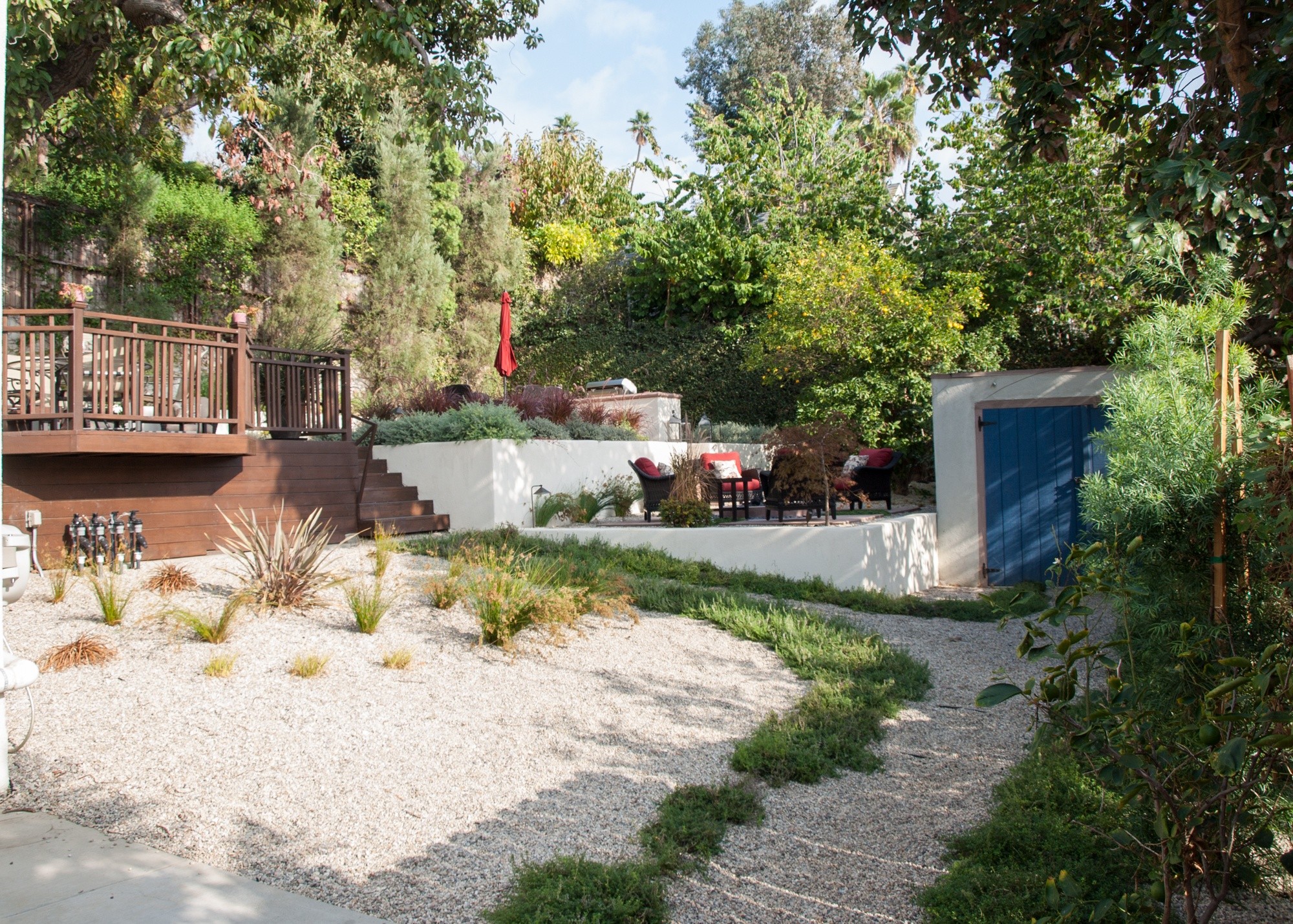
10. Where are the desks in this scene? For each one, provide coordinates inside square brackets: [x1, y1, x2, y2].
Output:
[6, 357, 152, 428]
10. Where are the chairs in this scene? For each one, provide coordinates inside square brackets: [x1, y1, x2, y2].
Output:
[829, 448, 901, 510]
[629, 458, 697, 523]
[6, 334, 207, 431]
[759, 448, 837, 523]
[698, 452, 762, 521]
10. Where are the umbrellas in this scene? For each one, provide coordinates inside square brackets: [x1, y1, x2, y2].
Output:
[493, 289, 518, 398]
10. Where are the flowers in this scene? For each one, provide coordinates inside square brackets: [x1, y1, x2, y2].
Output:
[59, 280, 94, 305]
[224, 295, 272, 329]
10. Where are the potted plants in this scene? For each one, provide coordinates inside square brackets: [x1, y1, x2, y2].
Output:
[171, 284, 367, 440]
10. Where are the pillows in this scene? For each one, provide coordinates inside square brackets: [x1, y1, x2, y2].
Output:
[656, 462, 674, 476]
[841, 454, 869, 477]
[710, 460, 742, 478]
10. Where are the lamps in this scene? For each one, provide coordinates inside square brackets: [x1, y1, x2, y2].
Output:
[533, 485, 550, 496]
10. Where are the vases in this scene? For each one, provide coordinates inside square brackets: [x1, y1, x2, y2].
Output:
[70, 290, 84, 302]
[232, 312, 246, 323]
